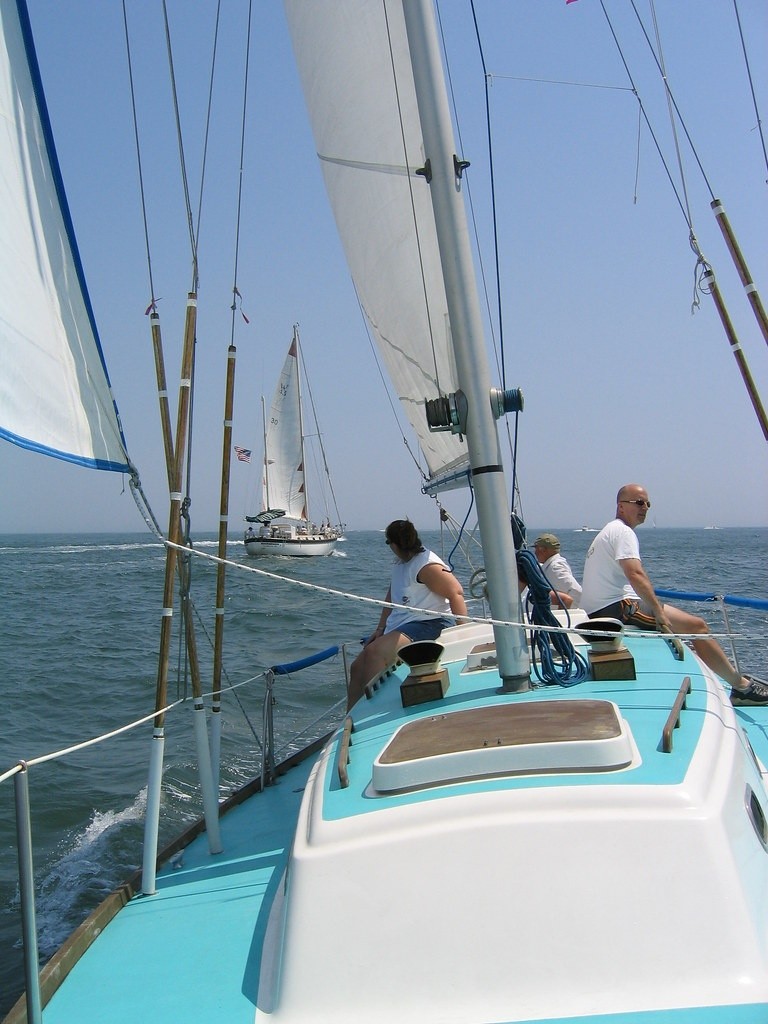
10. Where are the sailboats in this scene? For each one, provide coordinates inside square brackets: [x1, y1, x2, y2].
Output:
[244, 322, 346, 556]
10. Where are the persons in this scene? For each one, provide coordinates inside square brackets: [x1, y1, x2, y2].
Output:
[345, 520, 469, 713]
[579, 484, 768, 706]
[508, 514, 582, 614]
[247, 526, 256, 538]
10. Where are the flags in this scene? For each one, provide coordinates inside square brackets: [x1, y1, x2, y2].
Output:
[234, 446, 252, 463]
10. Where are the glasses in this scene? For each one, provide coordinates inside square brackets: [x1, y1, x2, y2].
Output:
[619, 500, 651, 508]
[386, 540, 392, 544]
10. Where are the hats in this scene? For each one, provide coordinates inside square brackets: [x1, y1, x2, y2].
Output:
[530, 532, 560, 550]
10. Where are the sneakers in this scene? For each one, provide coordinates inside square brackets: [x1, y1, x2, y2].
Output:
[729, 678, 768, 706]
